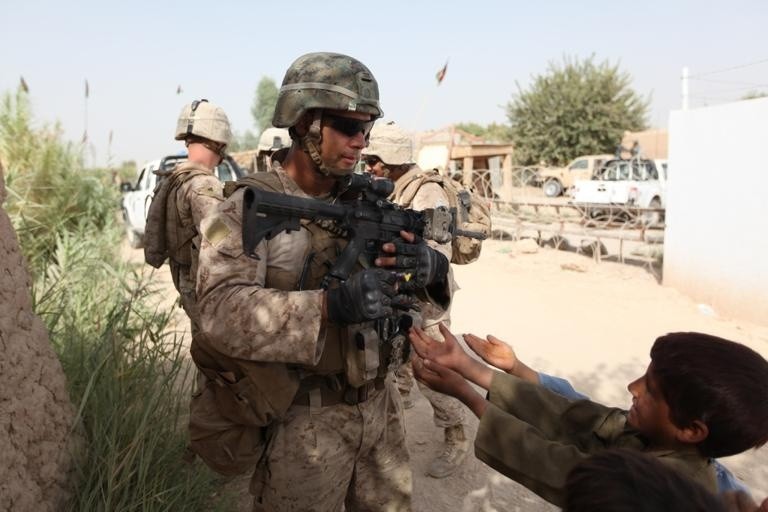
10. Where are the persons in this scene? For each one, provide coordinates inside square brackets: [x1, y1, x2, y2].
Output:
[561, 447, 721, 512]
[614, 138, 627, 160]
[193, 51, 451, 512]
[461, 329, 746, 491]
[630, 141, 640, 157]
[722, 489, 768, 512]
[144, 98, 232, 388]
[410, 271, 768, 512]
[363, 120, 490, 476]
[254, 126, 293, 173]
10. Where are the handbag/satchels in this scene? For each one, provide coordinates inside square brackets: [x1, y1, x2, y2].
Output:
[189, 333, 301, 476]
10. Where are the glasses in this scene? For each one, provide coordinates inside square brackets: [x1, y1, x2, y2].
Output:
[363, 158, 379, 167]
[314, 113, 375, 138]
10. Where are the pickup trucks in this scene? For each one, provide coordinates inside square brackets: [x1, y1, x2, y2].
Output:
[117, 152, 246, 250]
[567, 156, 668, 227]
[538, 153, 615, 198]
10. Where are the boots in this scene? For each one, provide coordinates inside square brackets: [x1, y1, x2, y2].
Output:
[401, 392, 414, 408]
[428, 424, 473, 478]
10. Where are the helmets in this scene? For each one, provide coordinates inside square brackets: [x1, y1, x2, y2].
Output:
[175, 98, 232, 146]
[256, 126, 293, 157]
[271, 52, 383, 130]
[361, 123, 417, 166]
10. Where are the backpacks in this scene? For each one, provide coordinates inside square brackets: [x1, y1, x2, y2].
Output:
[419, 166, 492, 265]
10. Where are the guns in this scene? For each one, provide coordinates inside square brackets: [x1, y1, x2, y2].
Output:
[240, 172, 489, 342]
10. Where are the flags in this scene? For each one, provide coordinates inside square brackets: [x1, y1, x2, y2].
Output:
[435, 56, 450, 84]
